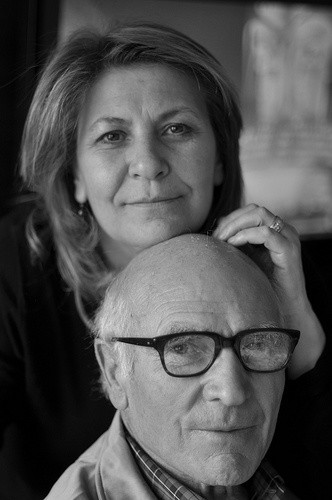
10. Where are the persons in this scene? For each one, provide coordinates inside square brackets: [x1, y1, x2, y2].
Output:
[0, 22, 332, 500]
[43, 233, 300, 500]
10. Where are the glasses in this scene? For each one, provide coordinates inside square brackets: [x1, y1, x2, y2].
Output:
[109, 328, 301, 377]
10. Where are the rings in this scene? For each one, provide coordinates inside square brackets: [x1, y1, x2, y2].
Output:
[269, 216, 286, 233]
